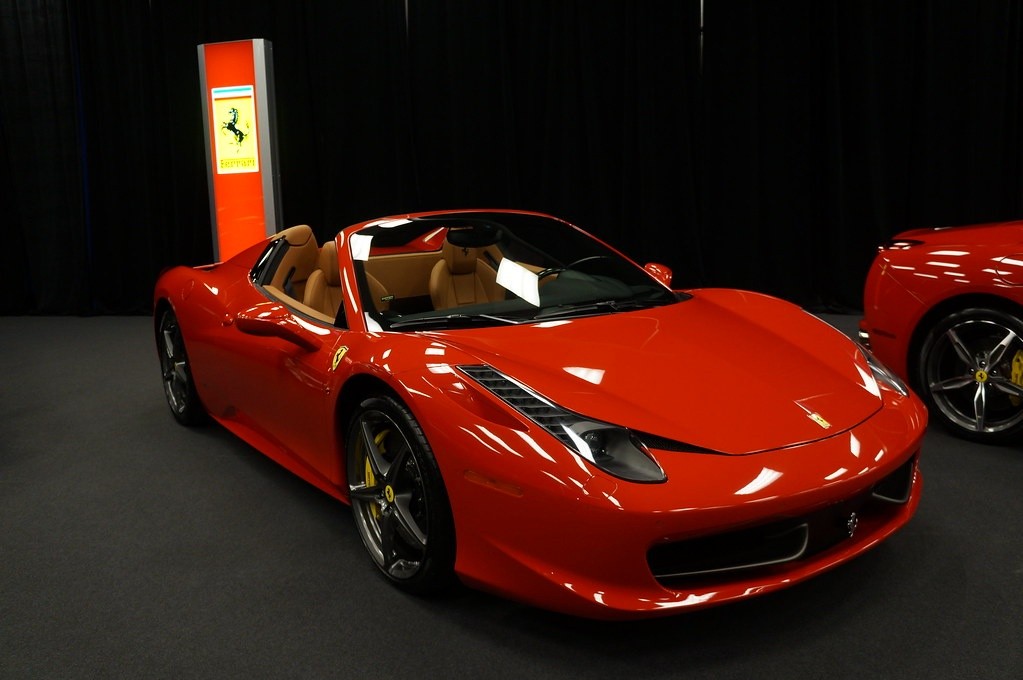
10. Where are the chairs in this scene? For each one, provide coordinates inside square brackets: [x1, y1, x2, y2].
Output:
[303, 241, 346, 318]
[429, 230, 506, 311]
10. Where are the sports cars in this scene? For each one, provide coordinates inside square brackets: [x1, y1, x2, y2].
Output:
[858, 214, 1023, 443]
[154, 207, 931, 624]
